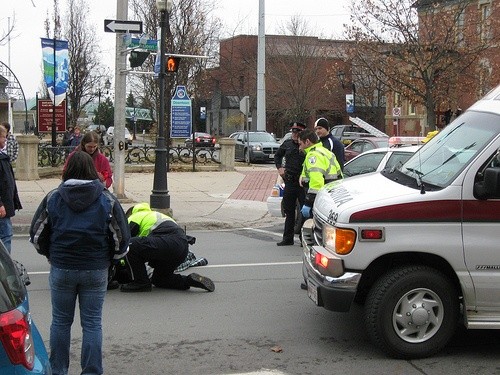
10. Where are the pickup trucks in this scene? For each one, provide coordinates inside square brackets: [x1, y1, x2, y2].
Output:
[331, 125, 373, 147]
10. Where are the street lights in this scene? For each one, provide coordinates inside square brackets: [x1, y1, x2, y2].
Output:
[98, 78, 110, 133]
[337, 66, 356, 118]
[149, 0, 174, 209]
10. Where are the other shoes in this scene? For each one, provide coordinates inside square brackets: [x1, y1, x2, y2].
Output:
[120, 282, 152, 291]
[277, 240, 295, 246]
[186, 273, 215, 292]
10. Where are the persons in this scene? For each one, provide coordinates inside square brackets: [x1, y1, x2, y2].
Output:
[0, 125, 23, 254]
[70, 127, 84, 146]
[63, 131, 112, 189]
[29, 150, 130, 375]
[314, 117, 344, 172]
[273, 121, 308, 247]
[62, 126, 74, 158]
[0, 122, 19, 162]
[296, 129, 344, 219]
[107, 203, 215, 293]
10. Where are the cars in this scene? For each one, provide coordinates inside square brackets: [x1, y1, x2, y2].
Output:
[343, 137, 391, 163]
[183, 133, 216, 147]
[229, 133, 282, 164]
[268, 136, 497, 235]
[82, 125, 106, 135]
[106, 126, 133, 146]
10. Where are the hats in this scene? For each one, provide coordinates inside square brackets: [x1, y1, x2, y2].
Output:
[314, 117, 330, 130]
[289, 122, 306, 131]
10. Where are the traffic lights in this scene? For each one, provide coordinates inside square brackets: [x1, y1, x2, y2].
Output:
[164, 55, 182, 73]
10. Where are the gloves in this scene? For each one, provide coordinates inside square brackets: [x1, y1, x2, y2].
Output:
[300, 206, 311, 217]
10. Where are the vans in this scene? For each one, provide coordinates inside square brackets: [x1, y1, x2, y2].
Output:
[301, 80, 500, 360]
